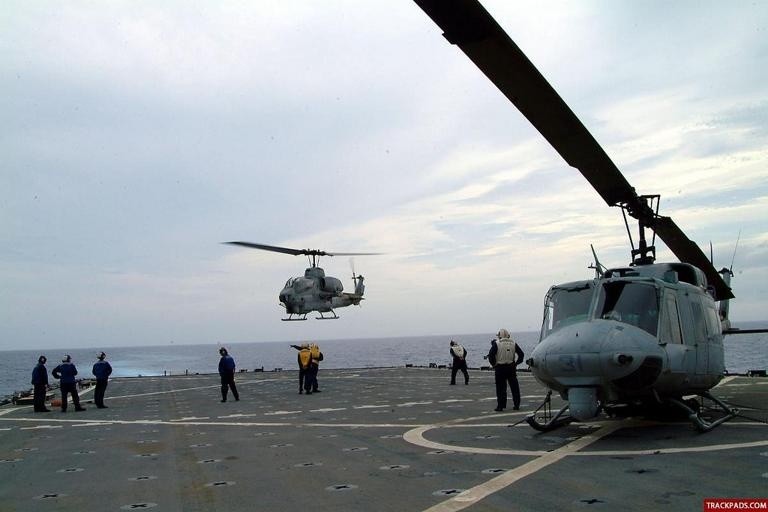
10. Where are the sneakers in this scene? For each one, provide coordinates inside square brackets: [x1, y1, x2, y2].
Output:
[298, 391, 303, 394]
[465, 381, 469, 385]
[311, 388, 322, 393]
[512, 406, 520, 410]
[96, 405, 108, 409]
[305, 390, 312, 394]
[74, 406, 86, 412]
[235, 398, 240, 401]
[61, 408, 67, 413]
[449, 382, 456, 385]
[34, 407, 51, 412]
[494, 407, 504, 411]
[221, 399, 227, 403]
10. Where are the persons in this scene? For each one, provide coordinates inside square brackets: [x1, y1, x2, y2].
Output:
[449, 340, 470, 385]
[92, 352, 112, 409]
[31, 356, 51, 412]
[52, 355, 86, 412]
[487, 329, 524, 411]
[290, 343, 323, 392]
[218, 347, 240, 402]
[298, 343, 312, 394]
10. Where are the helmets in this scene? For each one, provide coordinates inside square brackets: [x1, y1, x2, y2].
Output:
[450, 340, 458, 346]
[299, 341, 320, 351]
[496, 328, 510, 339]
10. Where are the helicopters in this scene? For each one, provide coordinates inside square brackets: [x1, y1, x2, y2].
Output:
[410, 0, 768, 433]
[220, 241, 386, 321]
